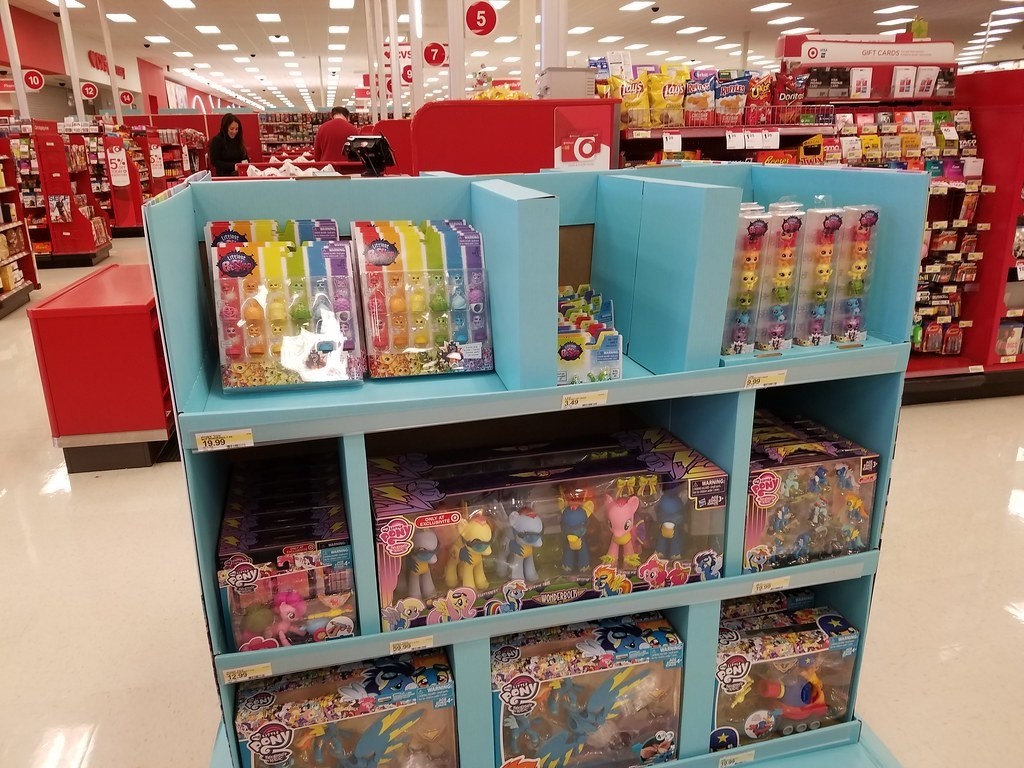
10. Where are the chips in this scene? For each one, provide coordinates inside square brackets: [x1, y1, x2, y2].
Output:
[688, 92, 743, 114]
[779, 110, 801, 123]
[628, 107, 684, 128]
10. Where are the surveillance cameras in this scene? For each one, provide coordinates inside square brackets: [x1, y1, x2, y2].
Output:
[0, 8, 337, 108]
[652, 7, 659, 12]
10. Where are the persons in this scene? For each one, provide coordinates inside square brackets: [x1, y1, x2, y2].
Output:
[314, 104, 362, 162]
[210, 113, 249, 176]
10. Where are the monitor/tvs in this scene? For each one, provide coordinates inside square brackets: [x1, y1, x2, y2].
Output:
[348, 136, 396, 177]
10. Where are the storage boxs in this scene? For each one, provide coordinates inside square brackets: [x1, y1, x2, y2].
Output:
[57, 118, 98, 133]
[36, 192, 45, 207]
[21, 124, 33, 133]
[218, 392, 881, 768]
[0, 117, 9, 127]
[996, 318, 1024, 355]
[23, 192, 36, 208]
[2, 203, 17, 222]
[163, 148, 182, 161]
[88, 136, 106, 164]
[31, 241, 52, 253]
[10, 125, 21, 133]
[0, 126, 9, 134]
[91, 181, 102, 193]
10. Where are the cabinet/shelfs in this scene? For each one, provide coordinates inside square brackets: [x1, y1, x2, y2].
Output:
[11, 117, 113, 270]
[0, 127, 41, 320]
[27, 264, 180, 474]
[131, 124, 167, 197]
[146, 164, 930, 768]
[260, 112, 380, 155]
[158, 127, 190, 187]
[84, 114, 145, 238]
